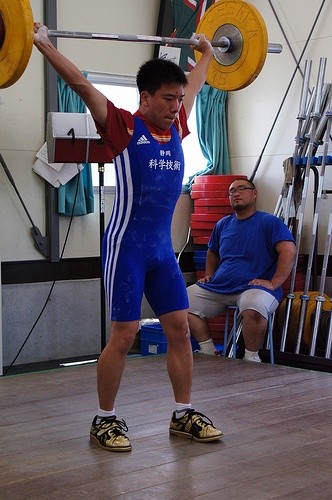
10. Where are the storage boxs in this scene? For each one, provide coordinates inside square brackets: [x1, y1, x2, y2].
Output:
[139, 324, 200, 356]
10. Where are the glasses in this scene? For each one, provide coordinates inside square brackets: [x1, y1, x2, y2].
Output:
[226, 185, 253, 196]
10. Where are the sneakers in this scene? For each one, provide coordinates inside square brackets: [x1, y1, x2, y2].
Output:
[169, 409, 222, 443]
[89, 415, 132, 452]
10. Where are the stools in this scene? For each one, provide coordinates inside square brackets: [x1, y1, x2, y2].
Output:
[224, 306, 273, 365]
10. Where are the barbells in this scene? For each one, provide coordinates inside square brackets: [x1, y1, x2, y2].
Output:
[0, 0, 283, 92]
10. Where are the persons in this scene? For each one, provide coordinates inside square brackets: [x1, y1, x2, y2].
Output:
[186, 178, 298, 362]
[32, 22, 224, 452]
